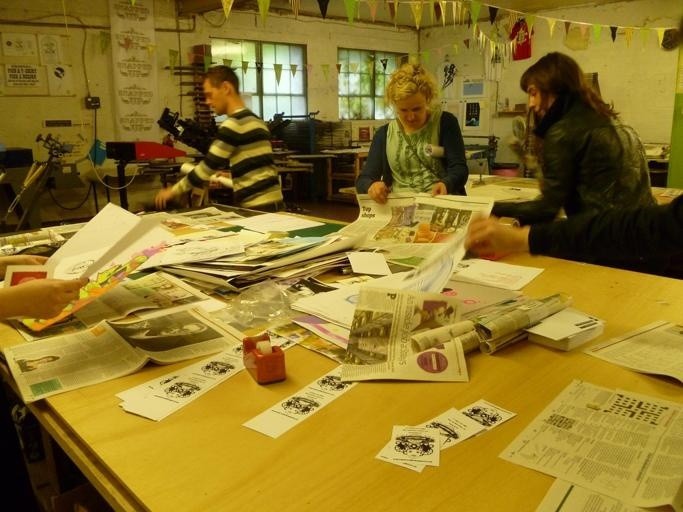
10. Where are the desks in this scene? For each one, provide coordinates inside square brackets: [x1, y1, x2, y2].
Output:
[287, 154, 336, 199]
[274, 160, 313, 212]
[321, 148, 368, 207]
[0, 204, 683, 512]
[338, 173, 682, 262]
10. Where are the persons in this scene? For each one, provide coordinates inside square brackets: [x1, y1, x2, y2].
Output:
[153, 283, 173, 294]
[16, 354, 61, 373]
[114, 318, 208, 350]
[0, 250, 90, 320]
[465, 193, 683, 282]
[354, 60, 469, 210]
[153, 64, 285, 214]
[415, 305, 453, 331]
[488, 48, 658, 219]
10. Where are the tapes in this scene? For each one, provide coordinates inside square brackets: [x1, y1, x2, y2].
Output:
[256, 340, 272, 356]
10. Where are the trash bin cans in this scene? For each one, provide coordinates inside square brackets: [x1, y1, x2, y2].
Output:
[491, 162, 520, 176]
